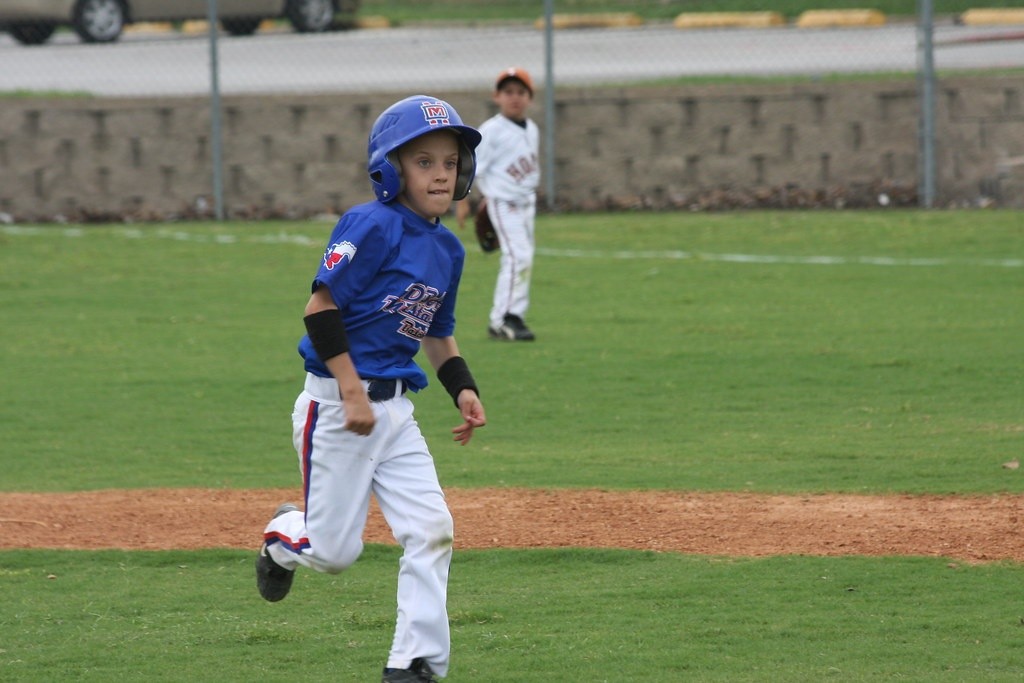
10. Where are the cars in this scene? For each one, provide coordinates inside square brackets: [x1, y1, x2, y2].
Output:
[1, 0, 359, 45]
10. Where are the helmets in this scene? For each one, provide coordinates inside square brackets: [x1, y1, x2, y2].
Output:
[368, 95, 482, 203]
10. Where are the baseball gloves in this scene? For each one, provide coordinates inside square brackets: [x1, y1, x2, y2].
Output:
[475, 204, 500, 251]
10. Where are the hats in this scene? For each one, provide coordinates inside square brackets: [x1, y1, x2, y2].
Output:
[496, 68, 535, 97]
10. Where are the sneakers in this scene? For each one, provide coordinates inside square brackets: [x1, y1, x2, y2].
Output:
[501, 314, 535, 342]
[256, 503, 300, 602]
[382, 658, 438, 683]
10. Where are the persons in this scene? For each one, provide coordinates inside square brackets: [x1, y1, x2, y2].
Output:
[455, 68, 539, 341]
[256, 96, 487, 683]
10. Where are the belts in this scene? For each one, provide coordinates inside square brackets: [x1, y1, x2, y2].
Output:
[366, 378, 408, 402]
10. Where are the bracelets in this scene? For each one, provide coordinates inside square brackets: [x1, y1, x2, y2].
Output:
[303, 309, 350, 361]
[436, 356, 480, 409]
